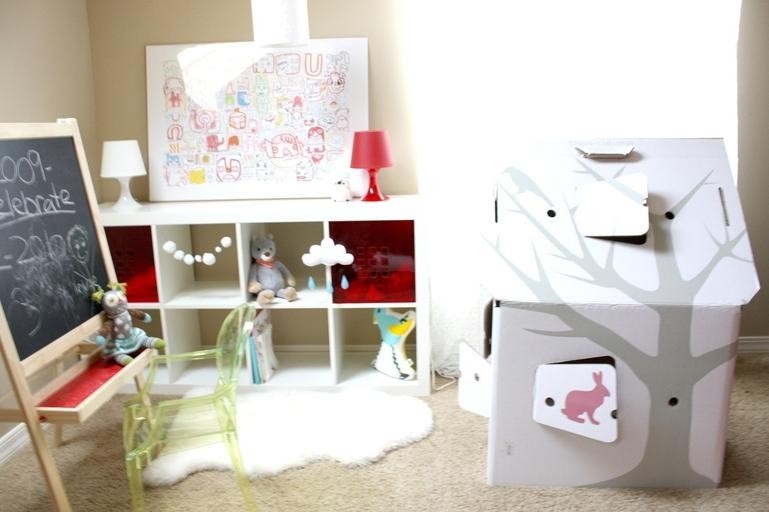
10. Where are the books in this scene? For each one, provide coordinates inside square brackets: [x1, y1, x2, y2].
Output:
[243, 309, 280, 385]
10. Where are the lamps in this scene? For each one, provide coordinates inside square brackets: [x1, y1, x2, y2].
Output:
[98, 140, 149, 206]
[351, 131, 391, 201]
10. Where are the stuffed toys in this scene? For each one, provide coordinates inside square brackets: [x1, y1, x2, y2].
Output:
[368, 308, 416, 380]
[90, 282, 166, 367]
[247, 233, 297, 308]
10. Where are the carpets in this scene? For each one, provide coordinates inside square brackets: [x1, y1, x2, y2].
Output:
[142, 388, 434, 486]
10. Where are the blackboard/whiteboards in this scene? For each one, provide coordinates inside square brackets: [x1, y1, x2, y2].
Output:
[145, 37, 369, 202]
[0, 118, 119, 378]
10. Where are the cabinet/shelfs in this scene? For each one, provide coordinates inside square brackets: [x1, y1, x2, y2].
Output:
[97, 196, 432, 399]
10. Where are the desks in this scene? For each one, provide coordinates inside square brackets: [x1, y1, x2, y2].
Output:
[485, 136, 762, 490]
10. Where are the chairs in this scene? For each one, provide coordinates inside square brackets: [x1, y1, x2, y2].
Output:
[120, 302, 252, 511]
